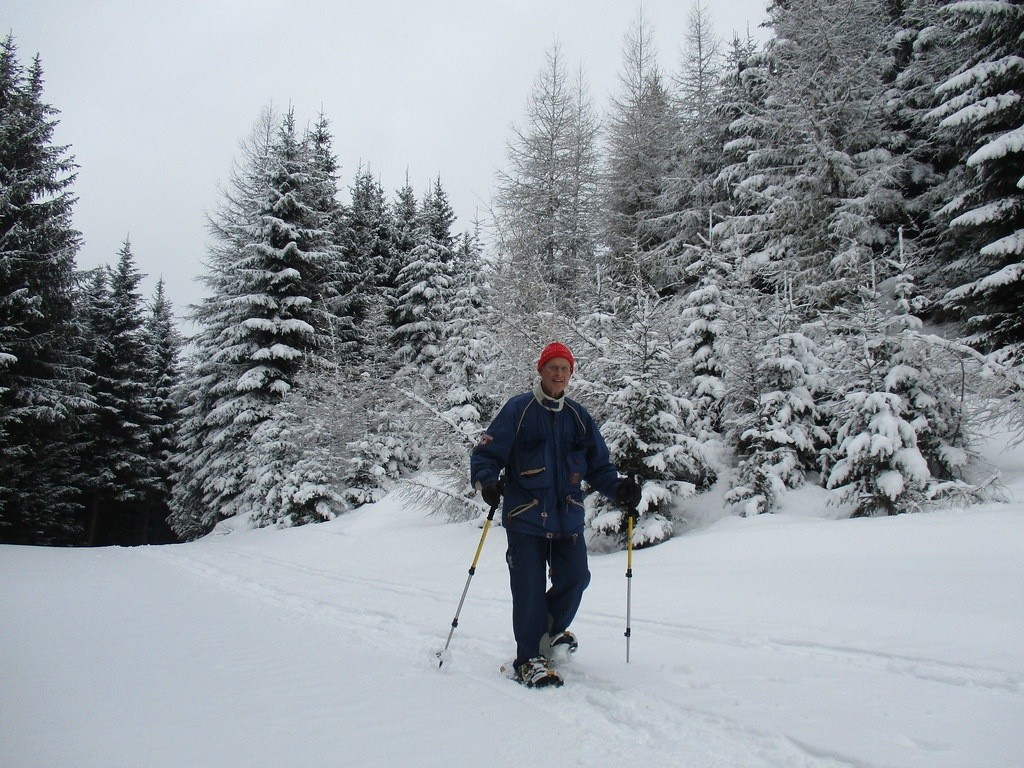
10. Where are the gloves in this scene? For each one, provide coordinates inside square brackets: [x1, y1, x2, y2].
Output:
[617, 478, 643, 513]
[482, 481, 505, 509]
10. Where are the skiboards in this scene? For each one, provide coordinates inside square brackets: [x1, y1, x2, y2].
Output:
[500, 629, 579, 688]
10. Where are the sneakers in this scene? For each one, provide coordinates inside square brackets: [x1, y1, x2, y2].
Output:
[512, 655, 563, 690]
[548, 630, 578, 665]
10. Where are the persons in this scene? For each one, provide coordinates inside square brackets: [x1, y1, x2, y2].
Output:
[469, 341, 642, 687]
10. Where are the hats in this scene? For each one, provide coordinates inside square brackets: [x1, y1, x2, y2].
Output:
[537, 342, 574, 376]
